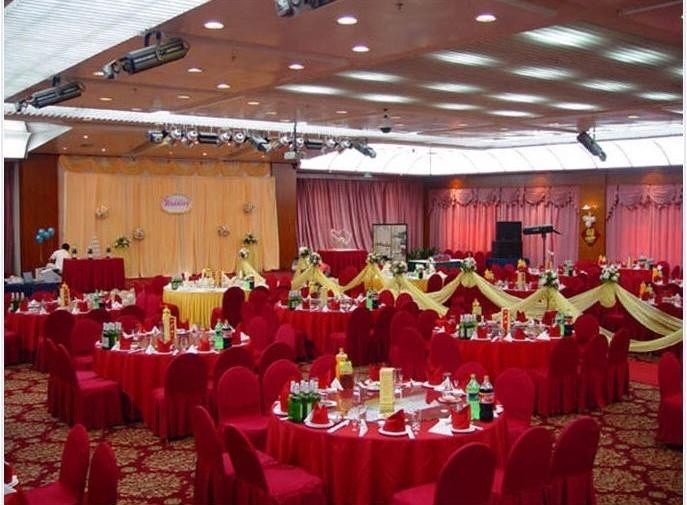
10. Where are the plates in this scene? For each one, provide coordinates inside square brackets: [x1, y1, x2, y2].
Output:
[451, 425, 476, 434]
[378, 426, 407, 436]
[305, 418, 335, 429]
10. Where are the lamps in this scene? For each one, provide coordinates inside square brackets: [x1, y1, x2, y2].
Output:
[14, 73, 85, 113]
[577, 123, 607, 162]
[144, 122, 377, 161]
[276, 0, 336, 18]
[101, 28, 189, 79]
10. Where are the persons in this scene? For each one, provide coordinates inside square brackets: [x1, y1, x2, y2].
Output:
[46, 243, 69, 274]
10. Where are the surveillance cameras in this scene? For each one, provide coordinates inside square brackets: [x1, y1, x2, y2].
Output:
[380, 114, 393, 134]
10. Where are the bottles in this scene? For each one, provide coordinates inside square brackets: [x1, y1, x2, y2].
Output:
[479, 375, 495, 420]
[366, 288, 378, 310]
[465, 374, 481, 420]
[335, 347, 348, 380]
[287, 378, 322, 423]
[563, 309, 573, 337]
[11, 292, 24, 310]
[99, 291, 106, 310]
[458, 314, 476, 338]
[101, 321, 122, 349]
[222, 319, 231, 349]
[215, 318, 223, 349]
[555, 309, 563, 334]
[94, 289, 99, 309]
[287, 291, 302, 310]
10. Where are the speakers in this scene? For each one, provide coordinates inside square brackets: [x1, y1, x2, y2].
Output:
[492, 240, 523, 257]
[495, 221, 522, 242]
